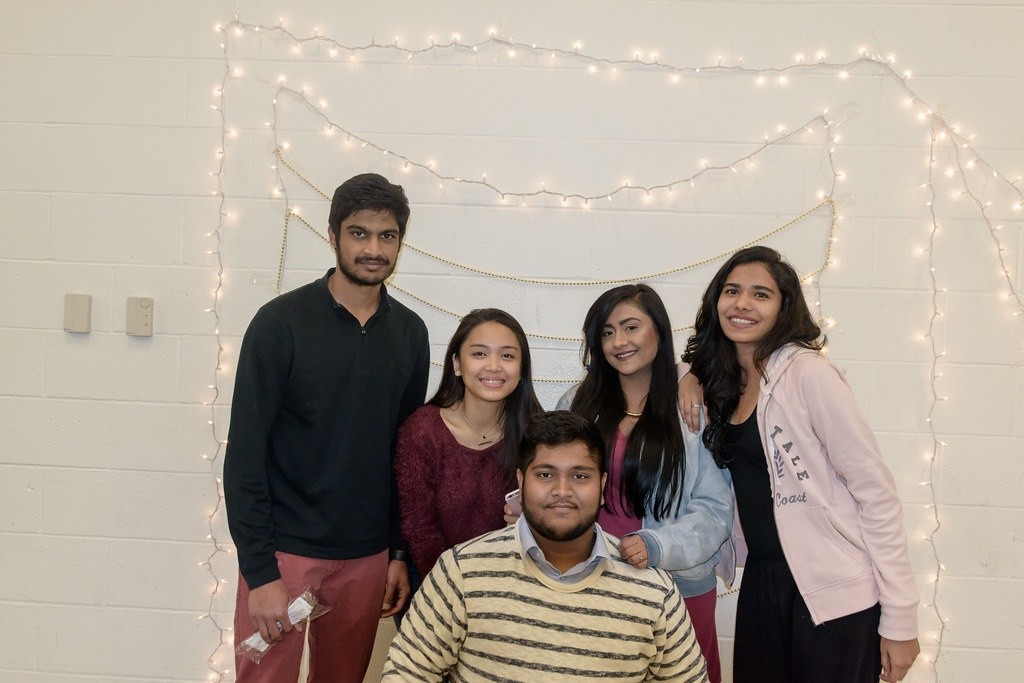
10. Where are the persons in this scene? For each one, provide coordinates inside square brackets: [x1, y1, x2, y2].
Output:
[504, 283, 737, 683]
[380, 410, 712, 683]
[677, 246, 923, 683]
[222, 174, 430, 683]
[391, 308, 546, 683]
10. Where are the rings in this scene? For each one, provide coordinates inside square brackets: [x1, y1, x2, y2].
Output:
[637, 554, 642, 563]
[691, 405, 702, 409]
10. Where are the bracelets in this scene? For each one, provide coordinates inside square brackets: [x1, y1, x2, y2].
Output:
[390, 550, 407, 561]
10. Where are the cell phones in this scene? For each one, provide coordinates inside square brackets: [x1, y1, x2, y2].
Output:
[505, 489, 523, 517]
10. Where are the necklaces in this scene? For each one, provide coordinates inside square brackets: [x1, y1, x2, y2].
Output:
[458, 404, 504, 439]
[626, 412, 644, 416]
